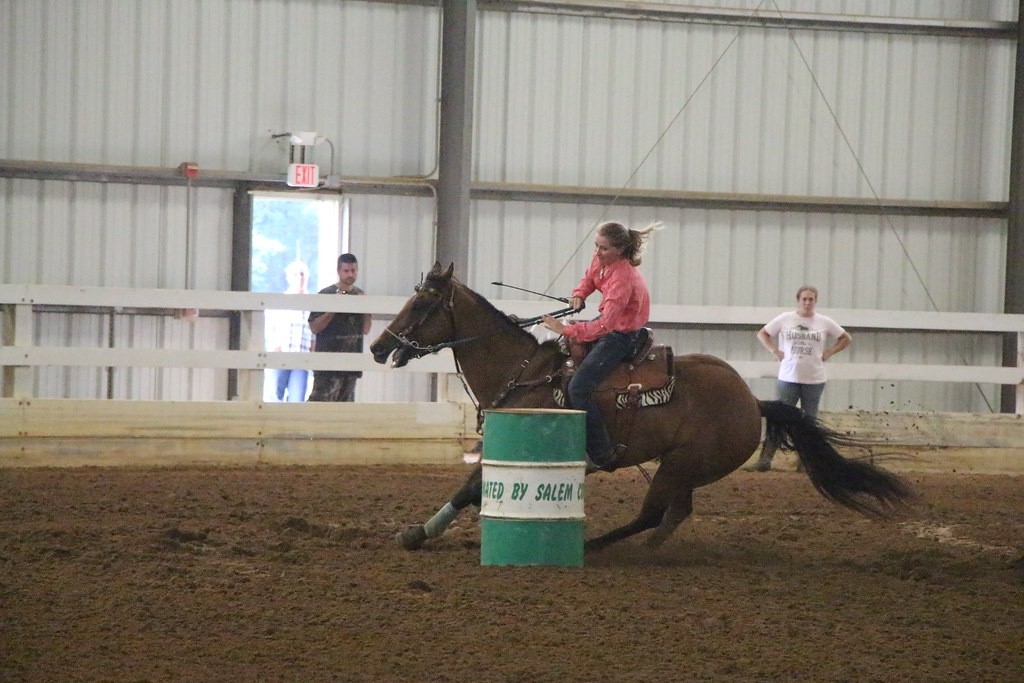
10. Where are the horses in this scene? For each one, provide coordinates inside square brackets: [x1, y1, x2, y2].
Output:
[369, 261, 930, 566]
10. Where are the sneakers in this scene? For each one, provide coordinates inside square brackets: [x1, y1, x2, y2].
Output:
[583, 451, 618, 476]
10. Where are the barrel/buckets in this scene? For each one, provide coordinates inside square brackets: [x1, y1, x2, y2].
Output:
[481, 404, 588, 573]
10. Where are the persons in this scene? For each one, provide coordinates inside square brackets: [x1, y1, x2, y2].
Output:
[306, 255, 372, 403]
[540, 221, 658, 475]
[742, 287, 852, 470]
[262, 262, 317, 404]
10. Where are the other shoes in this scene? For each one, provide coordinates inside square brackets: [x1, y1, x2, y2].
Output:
[743, 460, 770, 472]
